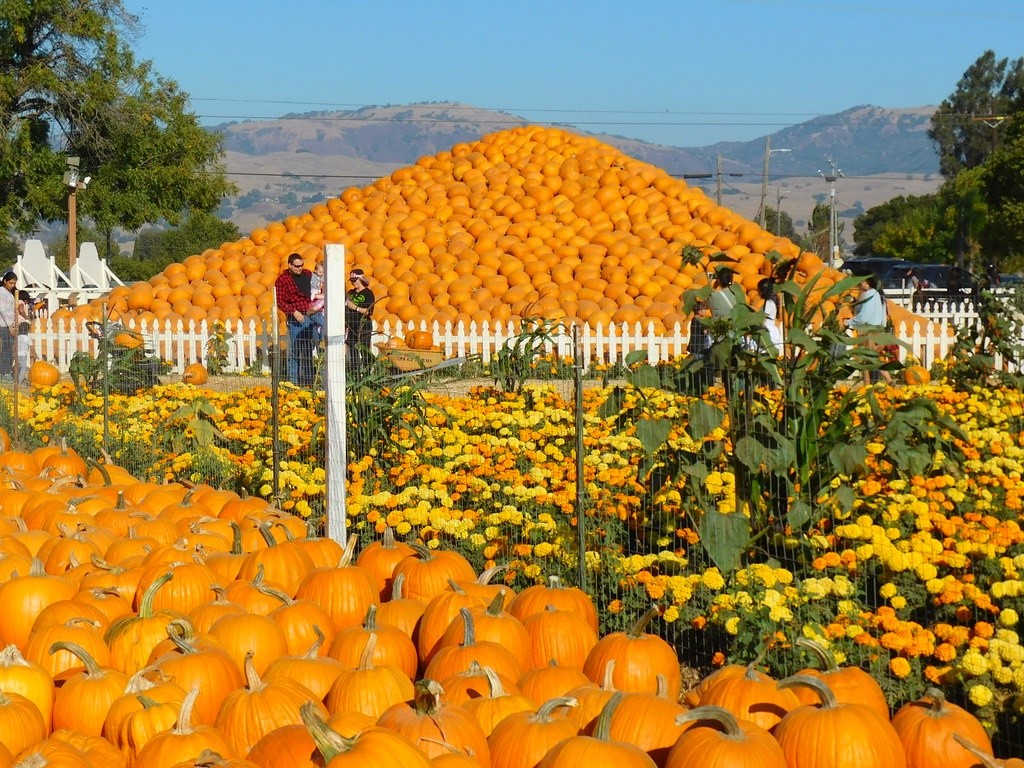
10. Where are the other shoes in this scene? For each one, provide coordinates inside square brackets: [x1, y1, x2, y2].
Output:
[2, 373, 14, 383]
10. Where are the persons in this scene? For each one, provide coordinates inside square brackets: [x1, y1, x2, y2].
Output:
[686, 263, 1022, 379]
[0, 272, 40, 385]
[274, 253, 375, 387]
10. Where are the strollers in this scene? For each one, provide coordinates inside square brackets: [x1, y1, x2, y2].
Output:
[86, 321, 162, 396]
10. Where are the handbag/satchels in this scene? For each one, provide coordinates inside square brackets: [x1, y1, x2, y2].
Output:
[8, 324, 19, 337]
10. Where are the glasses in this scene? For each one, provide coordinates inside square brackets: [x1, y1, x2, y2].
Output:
[291, 263, 304, 268]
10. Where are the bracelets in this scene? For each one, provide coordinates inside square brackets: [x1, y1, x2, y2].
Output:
[356, 306, 360, 311]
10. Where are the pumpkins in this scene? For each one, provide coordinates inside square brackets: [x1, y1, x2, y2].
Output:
[29, 124, 972, 388]
[0, 427, 1024, 768]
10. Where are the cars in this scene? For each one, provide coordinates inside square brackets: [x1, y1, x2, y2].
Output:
[838, 257, 1024, 295]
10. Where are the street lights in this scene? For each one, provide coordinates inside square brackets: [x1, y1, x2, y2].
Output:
[760, 149, 792, 230]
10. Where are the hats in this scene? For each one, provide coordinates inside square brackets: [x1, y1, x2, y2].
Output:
[348, 269, 364, 281]
